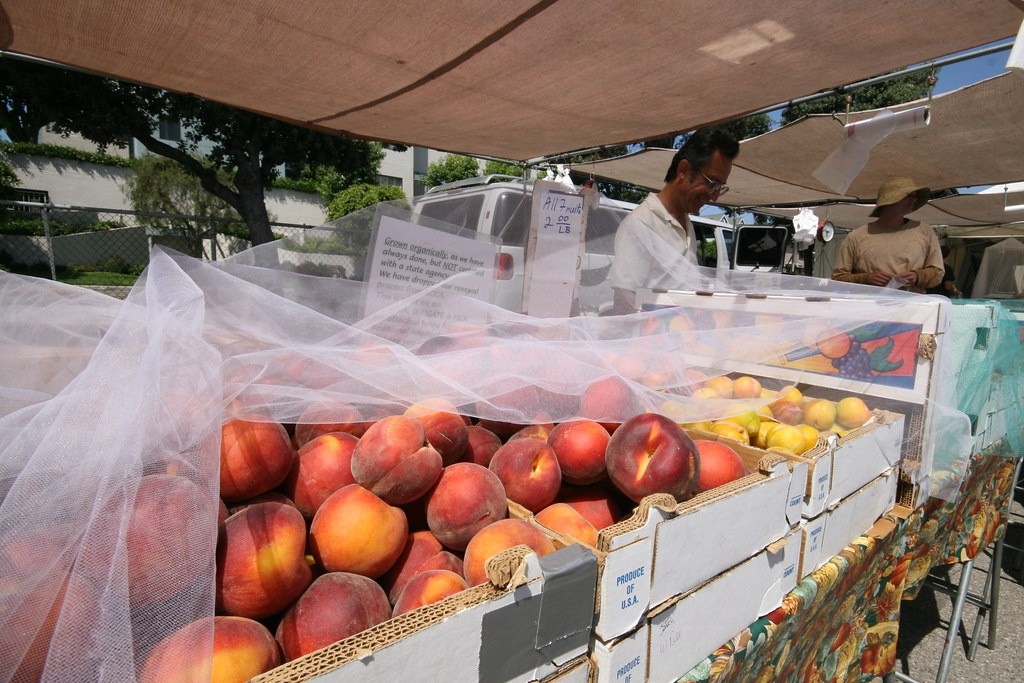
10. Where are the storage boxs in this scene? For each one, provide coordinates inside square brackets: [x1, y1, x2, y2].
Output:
[250, 286, 1024, 683]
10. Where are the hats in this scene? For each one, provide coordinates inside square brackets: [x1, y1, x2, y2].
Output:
[874, 174, 931, 217]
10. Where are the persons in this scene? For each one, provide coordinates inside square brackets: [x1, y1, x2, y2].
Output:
[926, 246, 960, 298]
[832, 176, 945, 285]
[609, 125, 740, 290]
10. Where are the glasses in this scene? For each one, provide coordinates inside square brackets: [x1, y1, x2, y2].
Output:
[697, 167, 729, 196]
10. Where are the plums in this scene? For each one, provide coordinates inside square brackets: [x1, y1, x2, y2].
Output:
[634, 369, 873, 457]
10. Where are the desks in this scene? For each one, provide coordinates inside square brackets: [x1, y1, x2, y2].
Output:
[679, 427, 1024, 683]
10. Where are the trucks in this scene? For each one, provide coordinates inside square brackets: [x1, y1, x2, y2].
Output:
[412, 173, 789, 318]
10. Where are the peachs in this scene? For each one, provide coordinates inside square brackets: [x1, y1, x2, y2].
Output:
[0, 359, 748, 683]
